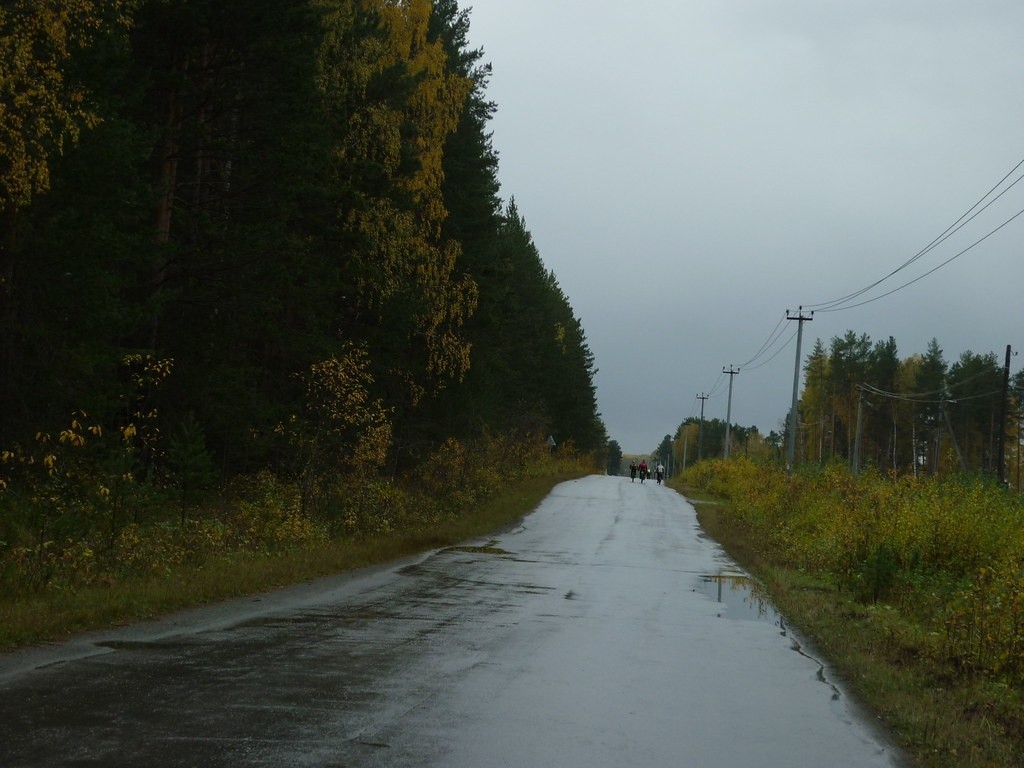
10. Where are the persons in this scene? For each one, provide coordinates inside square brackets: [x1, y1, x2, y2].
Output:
[630, 459, 668, 484]
